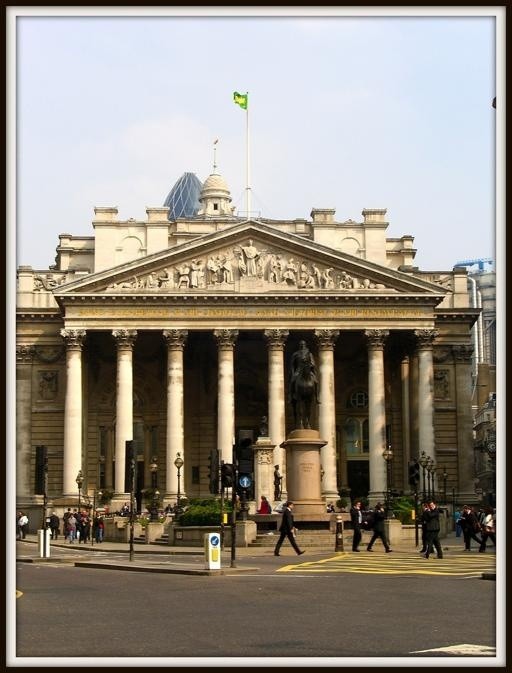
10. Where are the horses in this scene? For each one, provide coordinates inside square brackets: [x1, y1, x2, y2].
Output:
[291, 352, 316, 429]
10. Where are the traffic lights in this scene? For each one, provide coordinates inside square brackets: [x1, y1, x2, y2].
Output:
[221, 465, 230, 485]
[241, 434, 250, 473]
[411, 462, 421, 485]
[208, 454, 210, 481]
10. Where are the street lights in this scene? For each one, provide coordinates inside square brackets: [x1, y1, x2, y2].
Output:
[147, 459, 163, 521]
[418, 450, 449, 504]
[175, 451, 183, 509]
[74, 470, 85, 512]
[382, 442, 395, 519]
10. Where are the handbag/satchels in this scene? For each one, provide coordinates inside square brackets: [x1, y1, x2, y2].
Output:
[472, 522, 482, 533]
[483, 526, 495, 534]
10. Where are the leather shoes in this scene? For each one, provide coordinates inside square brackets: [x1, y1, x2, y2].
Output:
[352, 548, 360, 552]
[367, 547, 374, 552]
[298, 550, 306, 555]
[385, 549, 392, 553]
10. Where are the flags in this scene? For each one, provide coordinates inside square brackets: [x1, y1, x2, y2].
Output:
[233, 91, 248, 108]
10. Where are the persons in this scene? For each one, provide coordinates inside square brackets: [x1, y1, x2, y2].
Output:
[367, 502, 393, 553]
[453, 503, 496, 553]
[131, 238, 355, 288]
[331, 504, 336, 512]
[287, 338, 322, 404]
[257, 495, 271, 515]
[274, 501, 306, 556]
[351, 500, 368, 551]
[273, 464, 283, 500]
[16, 502, 177, 544]
[326, 503, 332, 512]
[419, 503, 435, 552]
[423, 501, 443, 558]
